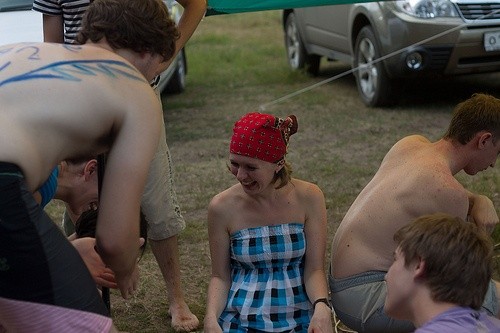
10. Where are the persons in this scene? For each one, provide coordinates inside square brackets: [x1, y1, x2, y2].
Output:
[203, 112, 334, 333]
[384, 212, 500, 333]
[0, 0, 206, 333]
[329, 93, 500, 333]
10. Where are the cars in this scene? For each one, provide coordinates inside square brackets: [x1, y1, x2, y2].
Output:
[0, 0, 208, 97]
[281, 0, 500, 108]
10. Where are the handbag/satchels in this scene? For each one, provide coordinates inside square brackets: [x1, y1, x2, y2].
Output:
[74, 209, 148, 237]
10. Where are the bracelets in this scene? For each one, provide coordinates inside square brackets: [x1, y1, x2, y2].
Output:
[313, 298, 332, 310]
